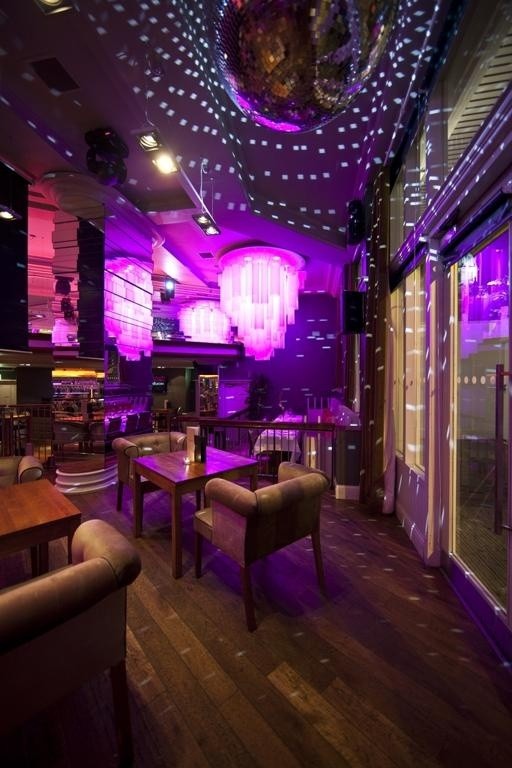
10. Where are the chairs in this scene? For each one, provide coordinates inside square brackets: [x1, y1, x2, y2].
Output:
[112, 430, 188, 514]
[257, 428, 265, 433]
[0, 455, 44, 486]
[247, 428, 273, 461]
[54, 421, 91, 456]
[89, 421, 105, 452]
[191, 459, 330, 633]
[1, 518, 144, 763]
[14, 410, 31, 456]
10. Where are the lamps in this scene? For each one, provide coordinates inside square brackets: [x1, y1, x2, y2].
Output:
[149, 153, 179, 175]
[62, 310, 75, 320]
[136, 132, 162, 152]
[82, 125, 135, 187]
[35, 0, 74, 20]
[53, 279, 71, 296]
[192, 212, 214, 228]
[201, 225, 221, 241]
[0, 206, 22, 221]
[204, 0, 400, 136]
[165, 278, 177, 300]
[61, 298, 74, 311]
[216, 252, 301, 362]
